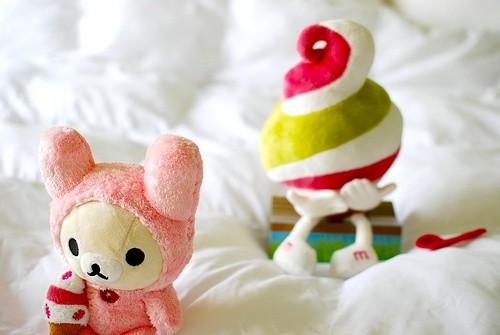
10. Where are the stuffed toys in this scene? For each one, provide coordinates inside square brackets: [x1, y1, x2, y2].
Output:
[32, 126, 204, 335]
[259, 18, 404, 277]
